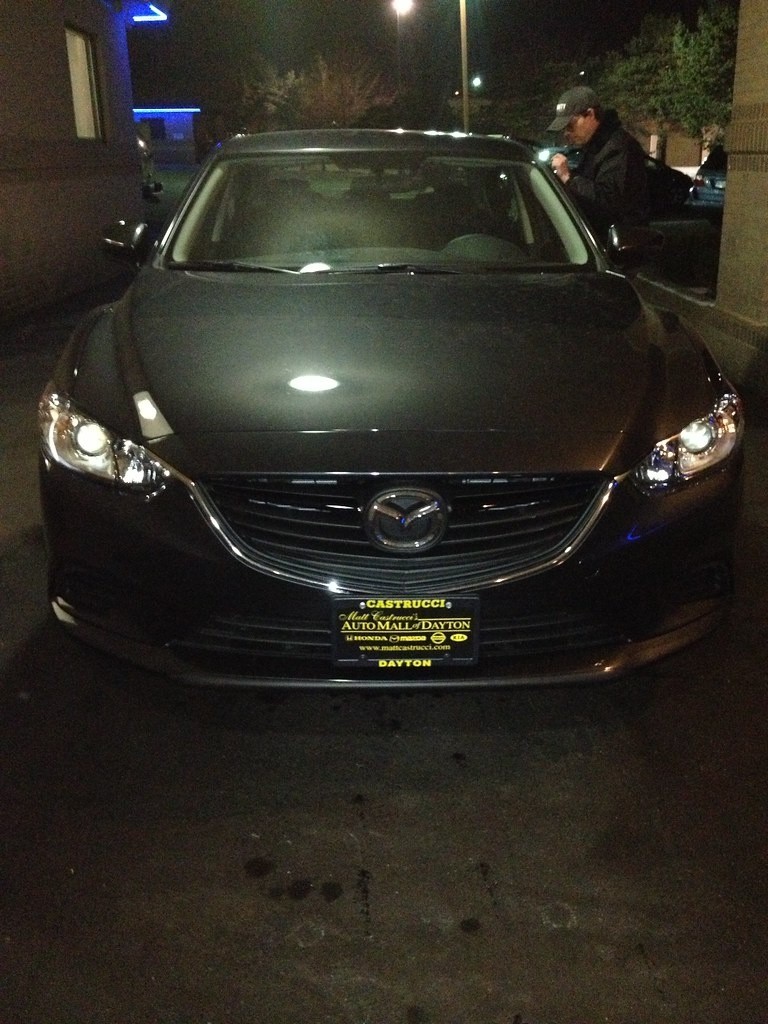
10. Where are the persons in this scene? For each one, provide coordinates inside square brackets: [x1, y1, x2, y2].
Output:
[543, 86, 651, 284]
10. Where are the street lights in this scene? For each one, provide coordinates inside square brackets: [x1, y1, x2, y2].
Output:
[387, 0, 472, 133]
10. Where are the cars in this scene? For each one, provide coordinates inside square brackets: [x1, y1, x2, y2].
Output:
[31, 128, 750, 701]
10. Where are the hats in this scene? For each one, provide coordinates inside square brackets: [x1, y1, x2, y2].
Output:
[545, 86, 600, 131]
